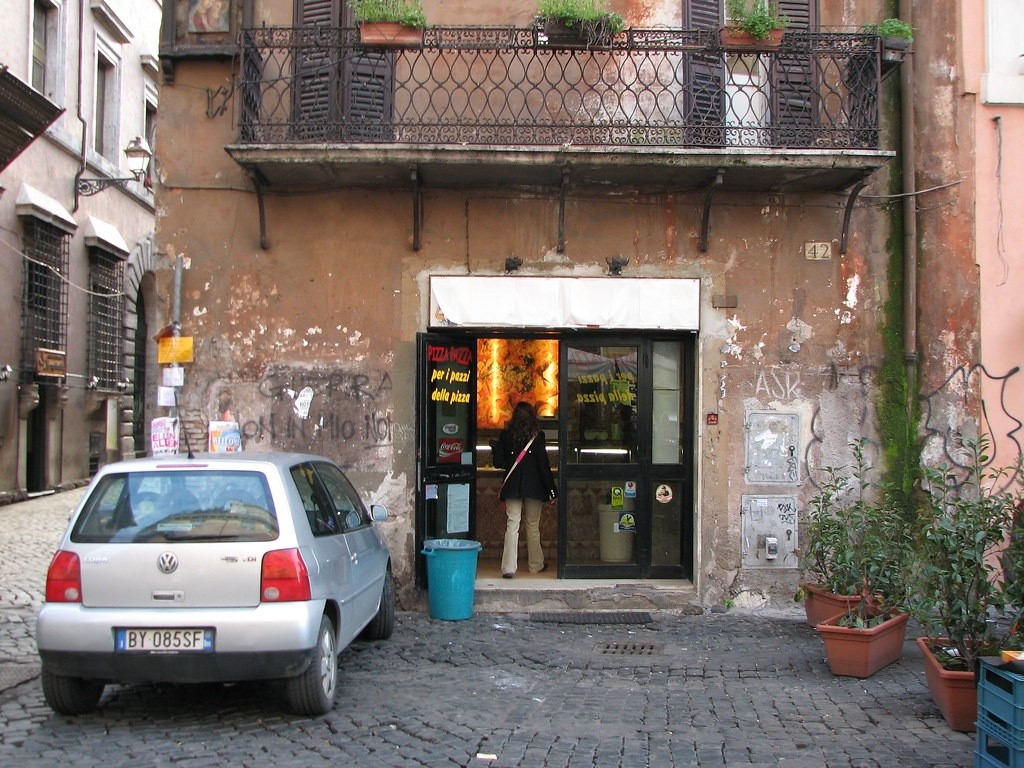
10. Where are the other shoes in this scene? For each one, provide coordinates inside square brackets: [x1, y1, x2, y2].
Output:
[540, 563, 548, 571]
[503, 574, 512, 578]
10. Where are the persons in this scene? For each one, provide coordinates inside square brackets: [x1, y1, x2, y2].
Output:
[489, 402, 558, 578]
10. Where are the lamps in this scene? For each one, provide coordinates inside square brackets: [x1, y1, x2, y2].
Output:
[606, 257, 631, 275]
[504, 256, 523, 271]
[77, 136, 153, 195]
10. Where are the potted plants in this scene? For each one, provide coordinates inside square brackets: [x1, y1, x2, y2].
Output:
[347, 0, 426, 47]
[716, 0, 790, 52]
[864, 17, 917, 60]
[538, 0, 625, 48]
[795, 434, 1024, 733]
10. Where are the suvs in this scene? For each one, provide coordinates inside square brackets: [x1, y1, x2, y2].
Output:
[36, 450, 394, 715]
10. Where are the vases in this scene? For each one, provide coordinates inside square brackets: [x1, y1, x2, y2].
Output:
[599, 502, 636, 563]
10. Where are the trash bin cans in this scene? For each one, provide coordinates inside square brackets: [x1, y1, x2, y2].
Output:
[599, 494, 635, 563]
[421, 539, 484, 621]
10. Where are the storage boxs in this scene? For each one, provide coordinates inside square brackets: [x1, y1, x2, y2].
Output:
[973, 660, 1024, 768]
